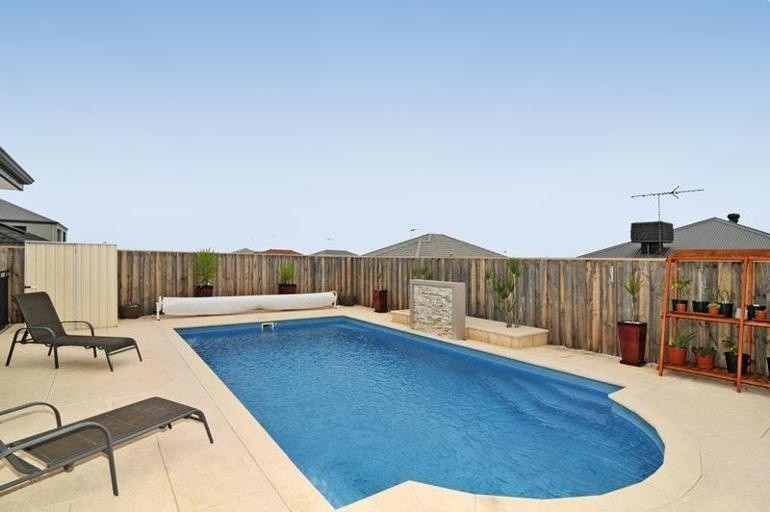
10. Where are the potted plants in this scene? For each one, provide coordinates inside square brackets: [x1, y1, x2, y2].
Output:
[372, 271, 388, 313]
[193, 248, 219, 296]
[277, 260, 296, 293]
[617, 267, 648, 367]
[666, 279, 770, 380]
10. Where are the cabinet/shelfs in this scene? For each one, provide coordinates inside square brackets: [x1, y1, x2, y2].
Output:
[658, 256, 769, 393]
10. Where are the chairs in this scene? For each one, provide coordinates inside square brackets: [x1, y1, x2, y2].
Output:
[1, 399, 215, 498]
[5, 291, 143, 373]
